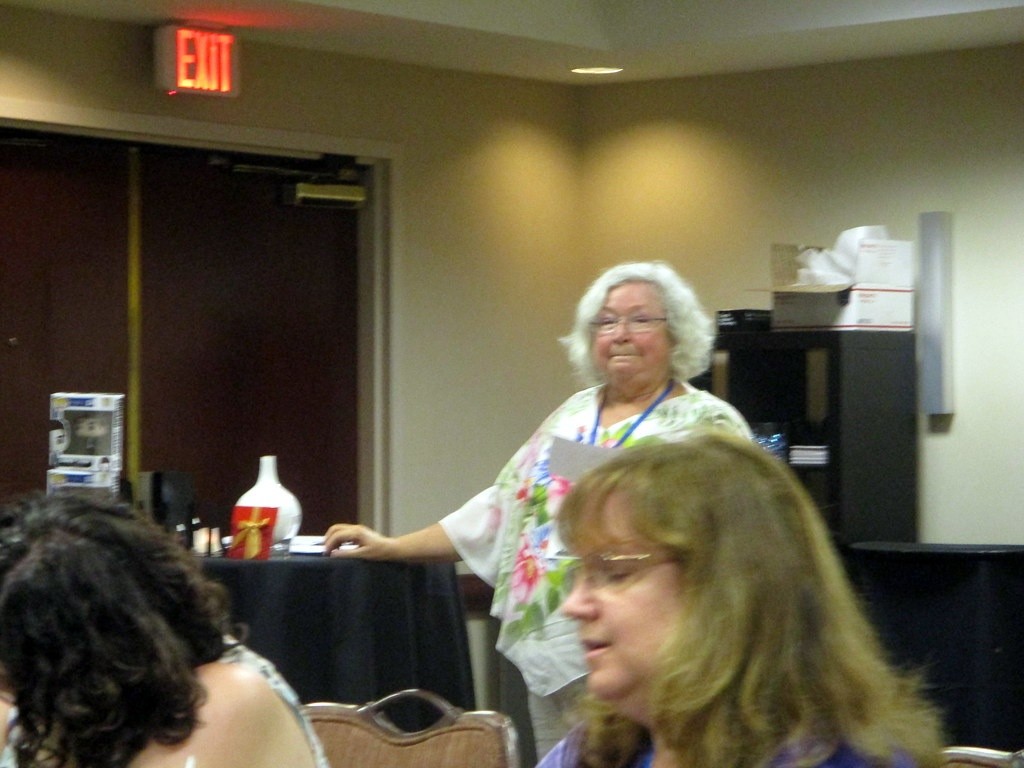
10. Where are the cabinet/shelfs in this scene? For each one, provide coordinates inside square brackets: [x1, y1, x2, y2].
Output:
[687, 330, 921, 547]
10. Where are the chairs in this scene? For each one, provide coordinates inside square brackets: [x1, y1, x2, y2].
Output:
[303, 686, 525, 768]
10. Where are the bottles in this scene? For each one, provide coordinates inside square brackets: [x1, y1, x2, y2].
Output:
[234, 455, 303, 551]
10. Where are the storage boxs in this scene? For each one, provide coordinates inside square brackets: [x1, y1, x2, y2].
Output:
[744, 237, 916, 332]
[47, 390, 126, 472]
[46, 469, 121, 498]
[715, 309, 772, 333]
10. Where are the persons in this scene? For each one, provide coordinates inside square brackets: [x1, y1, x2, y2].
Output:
[528, 436, 946, 768]
[0, 487, 330, 768]
[326, 260, 755, 760]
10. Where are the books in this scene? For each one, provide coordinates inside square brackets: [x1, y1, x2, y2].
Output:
[789, 445, 832, 464]
[289, 536, 362, 554]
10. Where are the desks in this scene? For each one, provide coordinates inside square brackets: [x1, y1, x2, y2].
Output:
[199, 553, 477, 733]
[844, 539, 1024, 754]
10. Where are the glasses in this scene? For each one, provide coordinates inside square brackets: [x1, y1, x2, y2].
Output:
[560, 550, 687, 596]
[589, 314, 672, 335]
